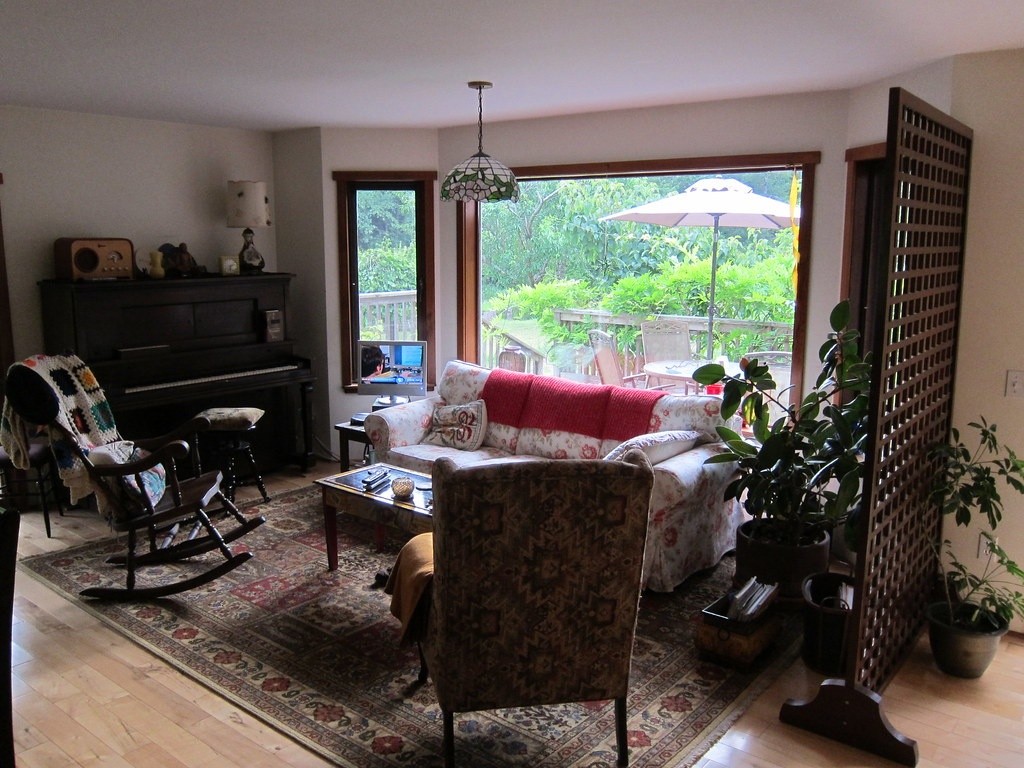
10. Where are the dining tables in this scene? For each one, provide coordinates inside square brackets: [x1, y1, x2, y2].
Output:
[642, 356, 744, 397]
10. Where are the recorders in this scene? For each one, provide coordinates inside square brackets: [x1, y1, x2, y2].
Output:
[54, 236, 136, 281]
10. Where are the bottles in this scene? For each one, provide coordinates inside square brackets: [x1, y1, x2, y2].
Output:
[499, 346, 526, 373]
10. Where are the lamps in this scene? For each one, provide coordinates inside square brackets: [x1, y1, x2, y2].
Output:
[224, 178, 275, 273]
[439, 81, 522, 204]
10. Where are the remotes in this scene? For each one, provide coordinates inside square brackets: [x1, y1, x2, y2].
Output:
[363, 467, 391, 490]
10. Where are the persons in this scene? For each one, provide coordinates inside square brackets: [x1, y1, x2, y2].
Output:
[361, 345, 412, 385]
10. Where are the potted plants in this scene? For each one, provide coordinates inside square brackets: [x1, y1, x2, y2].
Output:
[913, 414, 1024, 680]
[799, 499, 863, 679]
[691, 299, 875, 611]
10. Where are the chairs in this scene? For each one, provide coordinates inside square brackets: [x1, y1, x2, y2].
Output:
[395, 449, 656, 768]
[4, 343, 268, 602]
[586, 329, 676, 390]
[639, 319, 701, 395]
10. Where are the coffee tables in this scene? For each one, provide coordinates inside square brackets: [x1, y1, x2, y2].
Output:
[312, 462, 434, 570]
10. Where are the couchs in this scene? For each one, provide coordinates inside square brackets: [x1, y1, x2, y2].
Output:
[362, 359, 746, 595]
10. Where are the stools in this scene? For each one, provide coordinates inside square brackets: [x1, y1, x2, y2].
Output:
[192, 405, 274, 517]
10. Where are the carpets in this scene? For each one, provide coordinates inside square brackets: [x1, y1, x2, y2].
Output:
[15, 480, 803, 768]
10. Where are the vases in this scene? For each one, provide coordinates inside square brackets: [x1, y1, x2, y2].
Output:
[498, 346, 526, 372]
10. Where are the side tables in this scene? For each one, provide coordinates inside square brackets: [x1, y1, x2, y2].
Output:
[333, 421, 374, 474]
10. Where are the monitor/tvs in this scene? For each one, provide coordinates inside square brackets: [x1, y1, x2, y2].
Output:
[356, 340, 428, 396]
[389, 345, 423, 371]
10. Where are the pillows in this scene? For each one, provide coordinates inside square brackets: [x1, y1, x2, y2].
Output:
[416, 399, 488, 452]
[600, 429, 702, 467]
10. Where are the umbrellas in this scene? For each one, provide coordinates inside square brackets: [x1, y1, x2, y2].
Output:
[597, 178, 804, 363]
